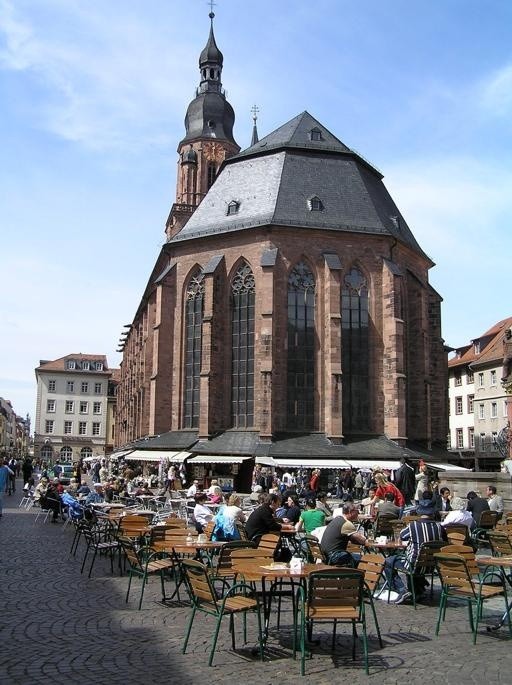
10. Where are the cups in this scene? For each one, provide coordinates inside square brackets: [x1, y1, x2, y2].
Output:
[199, 533, 207, 541]
[368, 529, 374, 543]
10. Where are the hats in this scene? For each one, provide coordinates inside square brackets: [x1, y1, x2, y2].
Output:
[416, 499, 435, 514]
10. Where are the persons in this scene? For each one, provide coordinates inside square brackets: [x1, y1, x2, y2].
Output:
[187, 478, 199, 512]
[373, 491, 399, 530]
[211, 493, 231, 542]
[415, 461, 428, 501]
[0, 454, 187, 527]
[207, 479, 225, 496]
[205, 487, 222, 504]
[316, 493, 333, 517]
[383, 499, 447, 604]
[224, 494, 246, 540]
[245, 493, 296, 556]
[360, 472, 405, 520]
[248, 493, 269, 517]
[440, 487, 452, 511]
[333, 469, 375, 505]
[467, 491, 493, 532]
[320, 502, 368, 568]
[282, 494, 301, 535]
[297, 497, 325, 551]
[251, 465, 322, 496]
[441, 497, 476, 535]
[193, 493, 214, 536]
[486, 484, 503, 521]
[394, 457, 416, 506]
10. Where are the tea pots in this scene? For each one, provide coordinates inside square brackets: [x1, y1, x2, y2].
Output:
[273, 537, 292, 563]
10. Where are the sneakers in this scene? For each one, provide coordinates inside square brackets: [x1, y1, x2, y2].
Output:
[394, 590, 412, 603]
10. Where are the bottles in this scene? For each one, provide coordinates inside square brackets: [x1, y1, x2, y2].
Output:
[187, 533, 192, 542]
